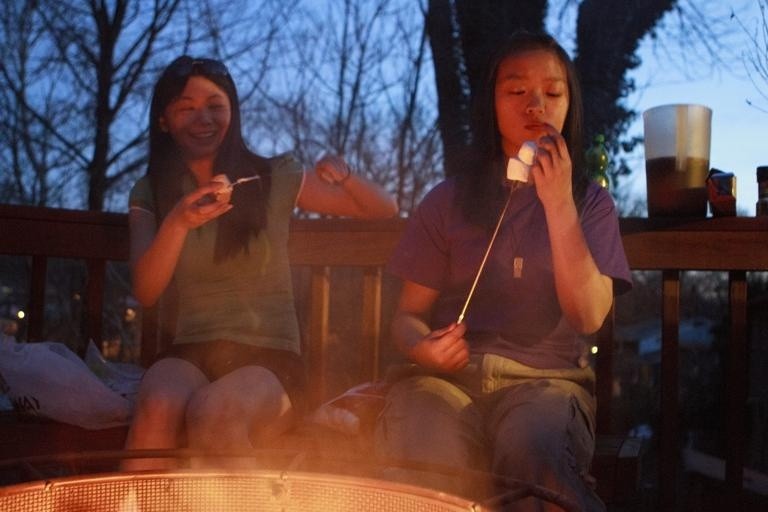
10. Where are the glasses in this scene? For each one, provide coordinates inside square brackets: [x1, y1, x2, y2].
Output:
[160, 57, 229, 79]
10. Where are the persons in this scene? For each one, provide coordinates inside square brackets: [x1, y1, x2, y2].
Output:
[116, 56, 400, 474]
[382, 32, 635, 511]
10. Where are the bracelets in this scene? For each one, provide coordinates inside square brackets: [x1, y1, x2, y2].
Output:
[335, 164, 352, 186]
